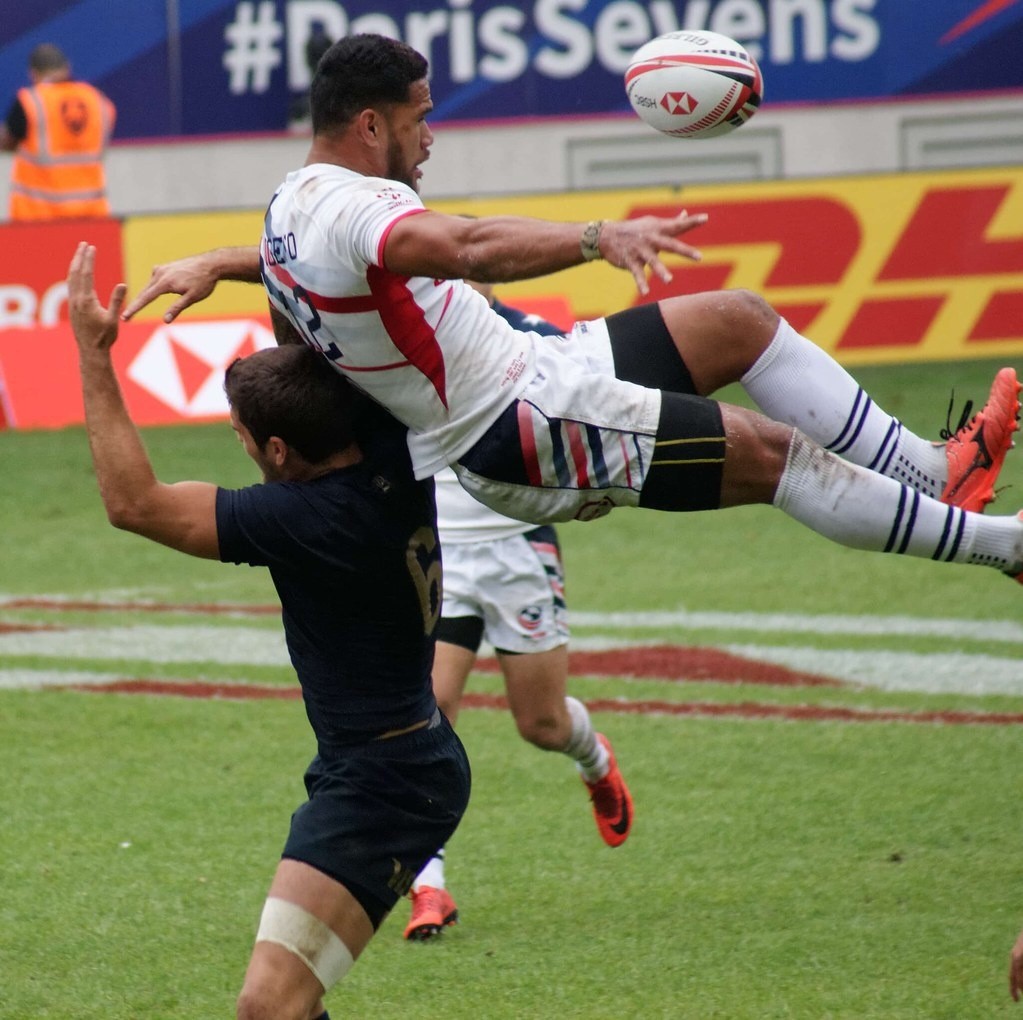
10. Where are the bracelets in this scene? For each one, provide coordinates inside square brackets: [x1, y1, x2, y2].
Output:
[579, 218, 605, 262]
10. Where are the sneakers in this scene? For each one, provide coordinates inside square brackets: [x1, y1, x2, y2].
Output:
[581, 733, 634, 847]
[932, 367, 1023, 513]
[405, 886, 457, 942]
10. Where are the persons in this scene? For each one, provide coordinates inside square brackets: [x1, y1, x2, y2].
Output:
[66, 241, 471, 1020]
[0, 43, 116, 223]
[260, 31, 1023, 582]
[398, 216, 635, 944]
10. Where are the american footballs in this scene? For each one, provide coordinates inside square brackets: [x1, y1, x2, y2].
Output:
[624, 30, 765, 138]
